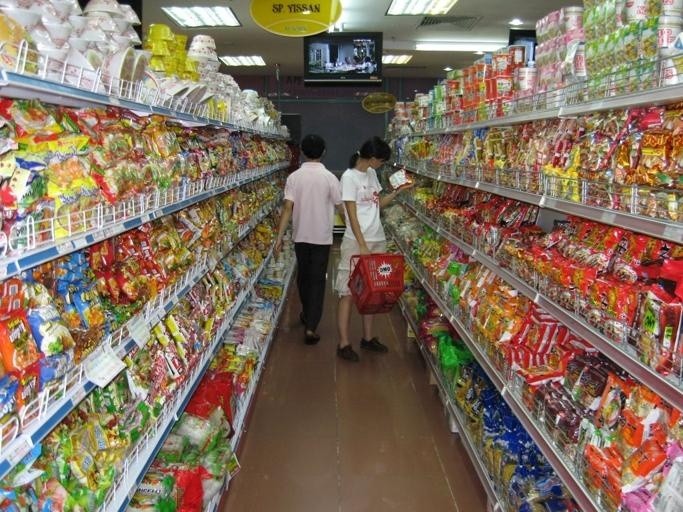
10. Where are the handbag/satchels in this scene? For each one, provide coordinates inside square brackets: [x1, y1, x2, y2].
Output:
[347, 253, 405, 314]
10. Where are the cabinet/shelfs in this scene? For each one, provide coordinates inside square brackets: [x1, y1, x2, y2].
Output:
[377, 51, 683, 512]
[1, 36, 296, 511]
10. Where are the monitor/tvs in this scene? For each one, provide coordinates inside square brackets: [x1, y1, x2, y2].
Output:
[508, 27, 537, 67]
[303, 31, 384, 87]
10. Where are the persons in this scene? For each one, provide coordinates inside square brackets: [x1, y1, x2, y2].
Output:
[331, 135, 417, 364]
[271, 132, 346, 345]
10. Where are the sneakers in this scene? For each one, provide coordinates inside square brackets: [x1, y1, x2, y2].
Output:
[360, 337, 387, 353]
[336, 344, 358, 361]
[300, 312, 308, 324]
[304, 335, 320, 345]
